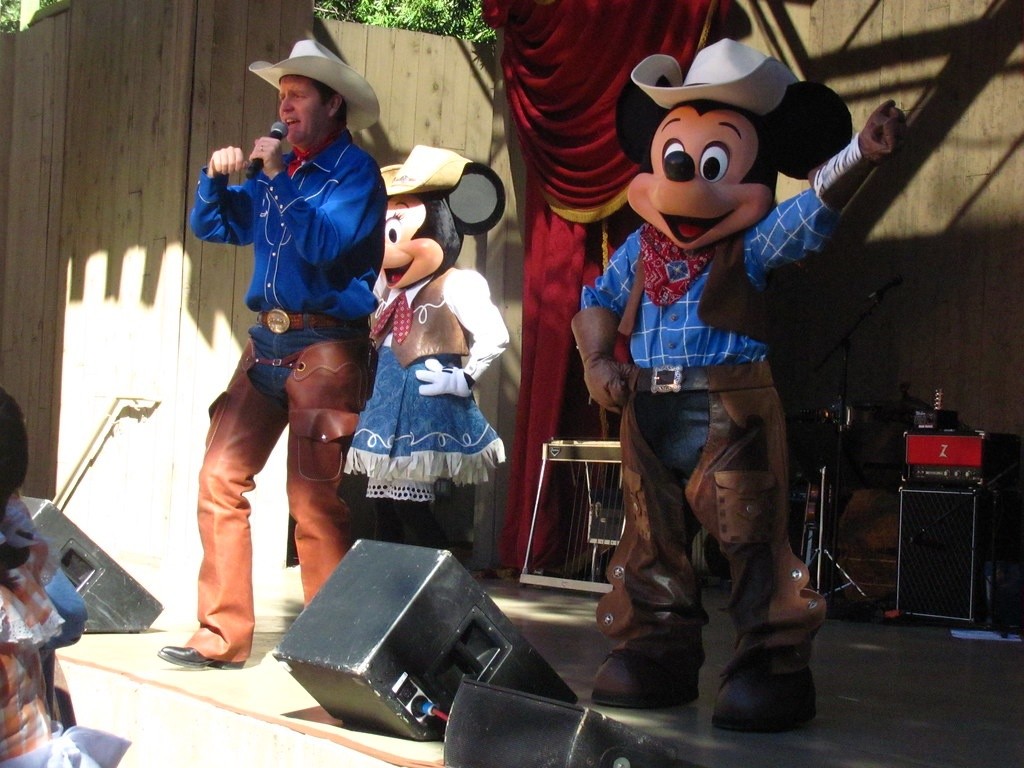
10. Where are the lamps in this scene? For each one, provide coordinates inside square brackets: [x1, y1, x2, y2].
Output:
[443, 673, 698, 768]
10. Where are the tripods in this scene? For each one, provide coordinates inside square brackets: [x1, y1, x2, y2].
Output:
[805, 467, 872, 609]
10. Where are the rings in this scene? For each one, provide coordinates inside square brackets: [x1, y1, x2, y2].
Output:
[261, 146, 263, 151]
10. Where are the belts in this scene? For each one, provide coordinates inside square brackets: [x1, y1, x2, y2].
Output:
[259, 309, 369, 334]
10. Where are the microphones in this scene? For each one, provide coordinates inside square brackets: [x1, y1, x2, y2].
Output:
[245, 122, 288, 180]
[868, 273, 904, 301]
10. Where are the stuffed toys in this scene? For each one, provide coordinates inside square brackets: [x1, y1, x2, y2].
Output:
[343, 144, 510, 567]
[572, 37, 906, 735]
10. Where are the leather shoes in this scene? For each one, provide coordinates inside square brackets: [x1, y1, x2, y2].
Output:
[158, 646, 245, 670]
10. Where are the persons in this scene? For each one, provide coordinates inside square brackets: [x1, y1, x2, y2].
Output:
[0, 386, 132, 768]
[155, 40, 387, 670]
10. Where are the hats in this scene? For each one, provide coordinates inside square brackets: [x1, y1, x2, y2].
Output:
[249, 40, 380, 131]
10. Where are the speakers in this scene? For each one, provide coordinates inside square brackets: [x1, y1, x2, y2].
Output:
[20, 498, 164, 632]
[272, 538, 701, 768]
[894, 483, 1024, 630]
